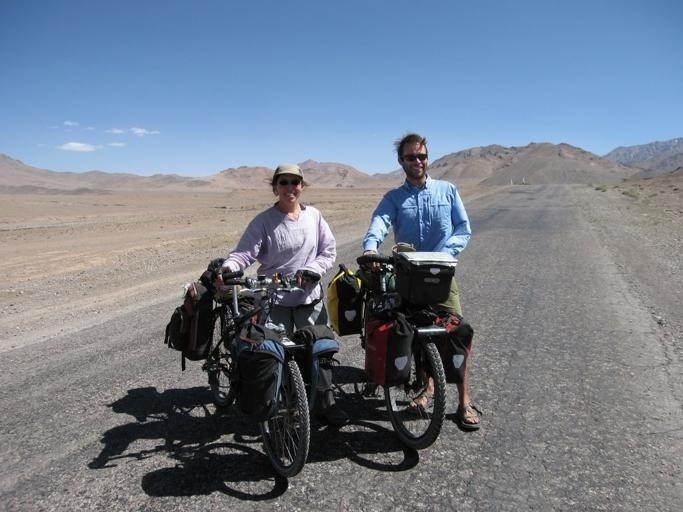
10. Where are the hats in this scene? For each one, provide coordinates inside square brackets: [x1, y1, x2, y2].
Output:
[275, 164, 305, 179]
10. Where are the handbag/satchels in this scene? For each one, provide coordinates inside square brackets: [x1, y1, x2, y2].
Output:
[433, 307, 475, 384]
[295, 324, 340, 411]
[361, 309, 413, 389]
[232, 324, 283, 424]
[326, 266, 365, 336]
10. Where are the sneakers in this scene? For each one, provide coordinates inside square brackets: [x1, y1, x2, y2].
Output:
[323, 406, 351, 425]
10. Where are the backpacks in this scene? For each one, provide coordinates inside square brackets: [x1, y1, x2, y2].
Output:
[164, 282, 215, 360]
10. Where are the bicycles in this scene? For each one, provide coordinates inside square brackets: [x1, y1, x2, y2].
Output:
[202, 271, 321, 479]
[356, 253, 458, 451]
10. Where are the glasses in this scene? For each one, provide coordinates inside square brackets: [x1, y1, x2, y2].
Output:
[401, 153, 427, 161]
[278, 178, 298, 185]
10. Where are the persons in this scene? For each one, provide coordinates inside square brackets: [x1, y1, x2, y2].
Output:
[361, 134, 479, 430]
[216, 164, 351, 425]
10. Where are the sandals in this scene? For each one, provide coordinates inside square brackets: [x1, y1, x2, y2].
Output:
[408, 391, 436, 412]
[457, 403, 481, 431]
